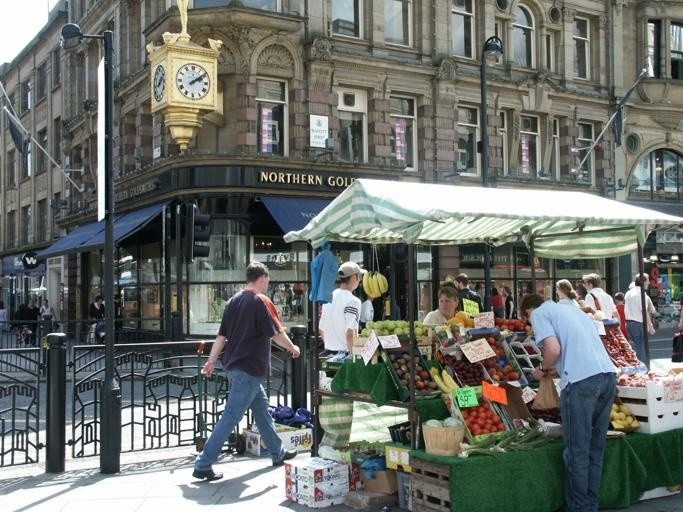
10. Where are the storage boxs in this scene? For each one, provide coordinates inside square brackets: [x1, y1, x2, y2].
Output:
[284, 458, 349, 509]
[510, 389, 537, 421]
[359, 468, 398, 495]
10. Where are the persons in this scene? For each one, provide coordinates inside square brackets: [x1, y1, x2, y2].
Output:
[553, 271, 656, 369]
[675, 280, 682, 334]
[455, 272, 514, 320]
[520, 293, 617, 512]
[11, 298, 57, 348]
[317, 261, 367, 357]
[191, 259, 300, 482]
[0, 299, 8, 350]
[114, 295, 124, 342]
[88, 295, 105, 321]
[422, 285, 459, 326]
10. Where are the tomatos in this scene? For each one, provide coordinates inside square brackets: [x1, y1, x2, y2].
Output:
[461, 401, 506, 436]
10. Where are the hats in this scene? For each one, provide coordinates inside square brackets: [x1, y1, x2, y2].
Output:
[338, 261, 367, 279]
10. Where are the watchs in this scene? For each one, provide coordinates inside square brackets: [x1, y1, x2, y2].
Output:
[538, 363, 547, 373]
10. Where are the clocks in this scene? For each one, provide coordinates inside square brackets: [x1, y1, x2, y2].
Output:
[153, 65, 166, 102]
[176, 63, 211, 101]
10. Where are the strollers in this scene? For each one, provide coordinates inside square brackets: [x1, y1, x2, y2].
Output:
[95, 318, 107, 352]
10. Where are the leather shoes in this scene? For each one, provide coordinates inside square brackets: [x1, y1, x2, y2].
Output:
[273, 449, 297, 466]
[192, 466, 223, 481]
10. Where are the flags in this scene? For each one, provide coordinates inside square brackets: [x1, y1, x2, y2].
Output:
[613, 78, 641, 149]
[7, 116, 27, 159]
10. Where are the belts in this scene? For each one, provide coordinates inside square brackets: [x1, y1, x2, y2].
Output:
[625, 319, 640, 324]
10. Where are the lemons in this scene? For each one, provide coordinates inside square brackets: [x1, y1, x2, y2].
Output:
[610, 403, 633, 425]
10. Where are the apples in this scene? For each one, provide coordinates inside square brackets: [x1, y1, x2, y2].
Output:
[361, 320, 424, 336]
[495, 317, 530, 332]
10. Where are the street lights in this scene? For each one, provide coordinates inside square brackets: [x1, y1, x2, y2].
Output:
[60, 21, 123, 476]
[480, 34, 506, 312]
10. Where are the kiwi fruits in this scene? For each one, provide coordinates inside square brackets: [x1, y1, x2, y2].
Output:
[389, 353, 437, 392]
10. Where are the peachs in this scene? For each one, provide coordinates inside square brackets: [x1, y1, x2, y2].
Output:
[481, 336, 519, 381]
